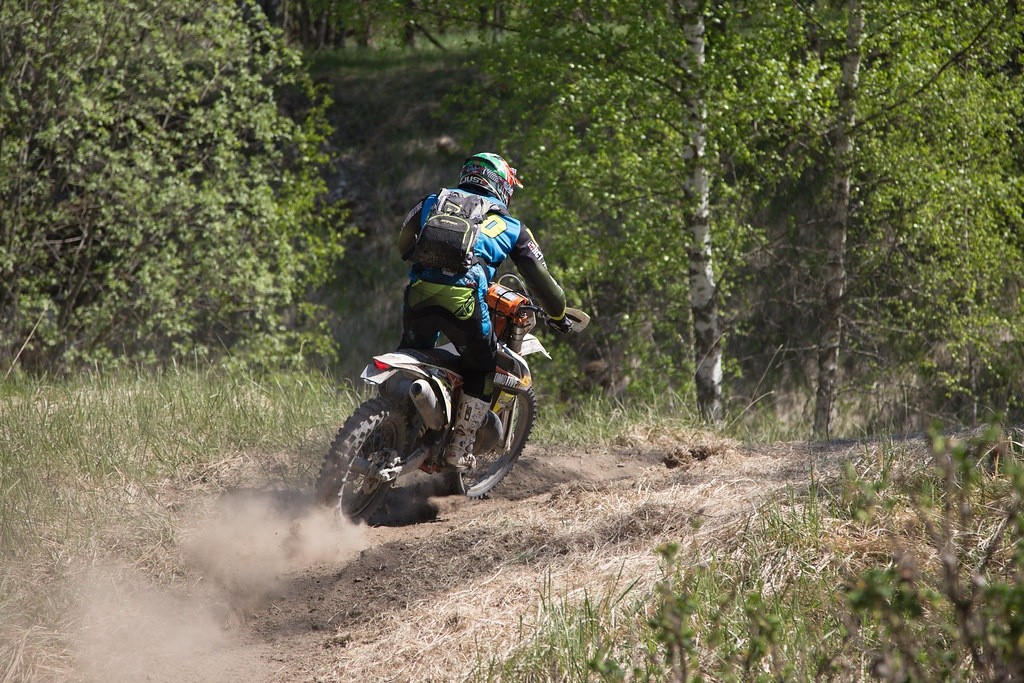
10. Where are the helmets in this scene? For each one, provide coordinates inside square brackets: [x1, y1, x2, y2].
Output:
[459, 153, 525, 209]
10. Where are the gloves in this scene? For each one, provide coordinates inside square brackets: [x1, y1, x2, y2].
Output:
[545, 314, 572, 334]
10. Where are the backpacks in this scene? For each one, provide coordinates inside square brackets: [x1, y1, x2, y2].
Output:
[413, 187, 510, 272]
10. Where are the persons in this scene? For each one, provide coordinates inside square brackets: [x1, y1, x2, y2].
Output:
[396, 151, 574, 474]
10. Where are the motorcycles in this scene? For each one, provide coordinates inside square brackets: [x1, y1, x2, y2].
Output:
[312, 271, 593, 526]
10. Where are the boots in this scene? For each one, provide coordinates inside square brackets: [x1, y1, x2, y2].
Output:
[445, 389, 493, 470]
